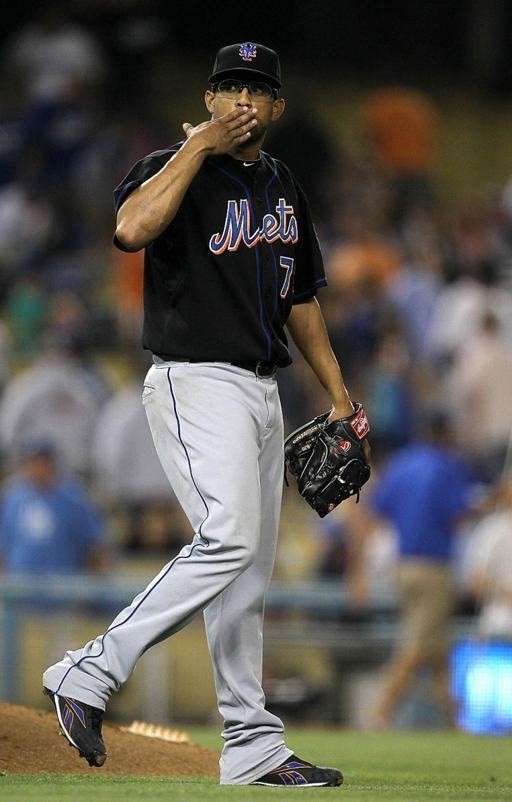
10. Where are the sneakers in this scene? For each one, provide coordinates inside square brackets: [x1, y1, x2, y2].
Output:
[248, 753, 346, 790]
[42, 683, 108, 769]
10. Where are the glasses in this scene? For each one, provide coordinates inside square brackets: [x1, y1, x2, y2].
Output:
[212, 77, 278, 97]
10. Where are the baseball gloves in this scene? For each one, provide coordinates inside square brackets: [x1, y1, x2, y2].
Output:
[283, 402, 374, 520]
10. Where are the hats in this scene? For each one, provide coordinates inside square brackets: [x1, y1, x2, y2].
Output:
[206, 41, 284, 91]
